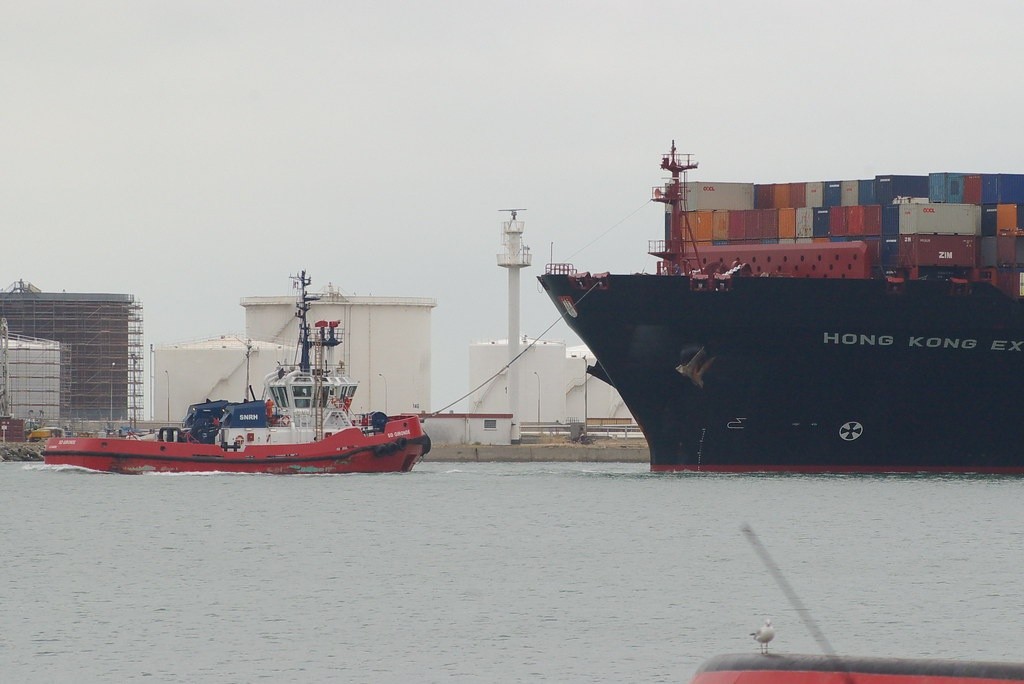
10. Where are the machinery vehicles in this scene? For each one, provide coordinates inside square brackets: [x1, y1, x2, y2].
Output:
[23, 421, 63, 442]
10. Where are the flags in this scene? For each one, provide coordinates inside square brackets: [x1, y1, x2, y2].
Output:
[213, 418, 219, 425]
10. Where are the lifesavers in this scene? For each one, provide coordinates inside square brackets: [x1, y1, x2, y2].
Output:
[281, 415, 291, 426]
[235, 435, 244, 445]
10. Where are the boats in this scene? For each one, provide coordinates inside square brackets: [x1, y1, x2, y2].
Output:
[536, 139, 1023, 478]
[42, 266, 434, 473]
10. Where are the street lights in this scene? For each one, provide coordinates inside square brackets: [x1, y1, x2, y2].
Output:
[166, 371, 170, 422]
[533, 372, 541, 425]
[378, 374, 387, 416]
[110, 361, 116, 420]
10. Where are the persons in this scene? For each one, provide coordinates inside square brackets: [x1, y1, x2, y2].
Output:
[345, 397, 350, 412]
[675, 264, 680, 275]
[731, 257, 742, 275]
[266, 399, 274, 416]
